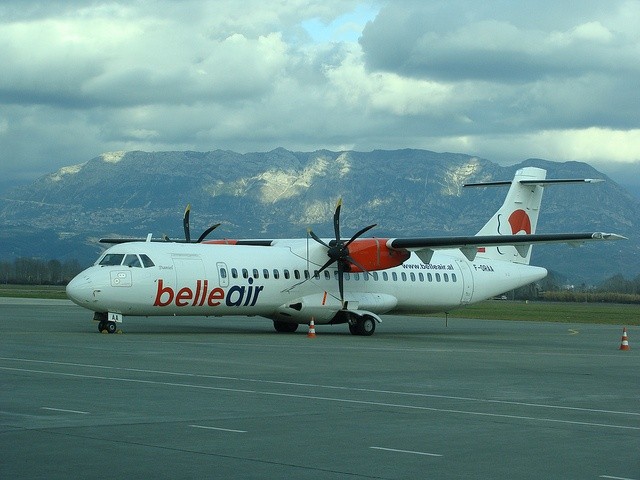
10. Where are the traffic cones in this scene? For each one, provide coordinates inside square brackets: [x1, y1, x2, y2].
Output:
[620, 327, 630, 350]
[307, 317, 318, 338]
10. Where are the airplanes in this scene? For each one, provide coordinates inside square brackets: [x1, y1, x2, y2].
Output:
[66, 167, 628, 336]
